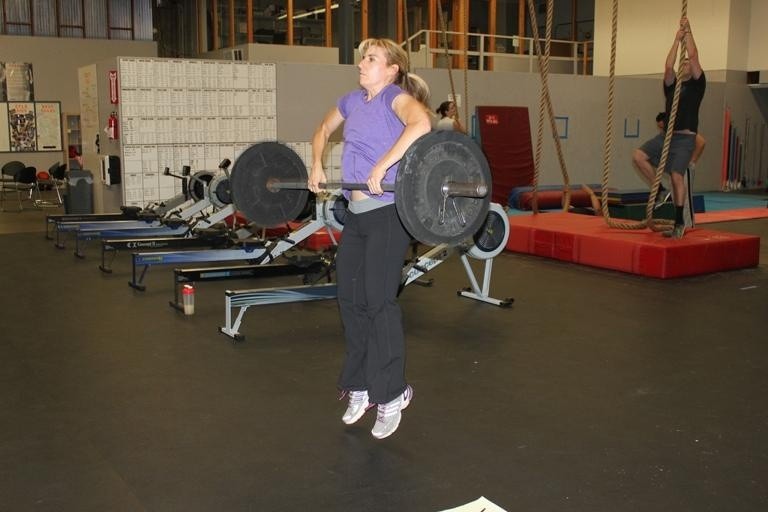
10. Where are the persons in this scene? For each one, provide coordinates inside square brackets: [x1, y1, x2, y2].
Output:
[632, 16, 706, 239]
[651, 112, 707, 229]
[308, 38, 436, 441]
[435, 100, 464, 132]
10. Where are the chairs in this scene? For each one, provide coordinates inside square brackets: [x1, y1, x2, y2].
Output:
[0, 160, 68, 212]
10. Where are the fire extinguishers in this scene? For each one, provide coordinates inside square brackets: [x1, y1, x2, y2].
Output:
[108, 112, 117, 140]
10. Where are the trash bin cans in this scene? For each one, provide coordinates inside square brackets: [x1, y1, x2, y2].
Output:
[64, 171, 93, 214]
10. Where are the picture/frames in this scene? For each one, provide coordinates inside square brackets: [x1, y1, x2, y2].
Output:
[0, 100, 64, 152]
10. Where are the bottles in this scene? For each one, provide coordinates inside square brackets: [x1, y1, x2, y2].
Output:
[182, 284, 195, 315]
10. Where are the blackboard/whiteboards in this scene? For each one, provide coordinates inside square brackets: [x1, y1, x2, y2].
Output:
[0, 101, 63, 152]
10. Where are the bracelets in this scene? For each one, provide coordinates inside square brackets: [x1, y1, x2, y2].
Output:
[683, 31, 692, 34]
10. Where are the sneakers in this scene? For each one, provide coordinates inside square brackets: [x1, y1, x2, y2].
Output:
[341, 388, 375, 425]
[370, 383, 414, 441]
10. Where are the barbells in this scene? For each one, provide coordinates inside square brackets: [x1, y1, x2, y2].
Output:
[229, 129, 493, 247]
